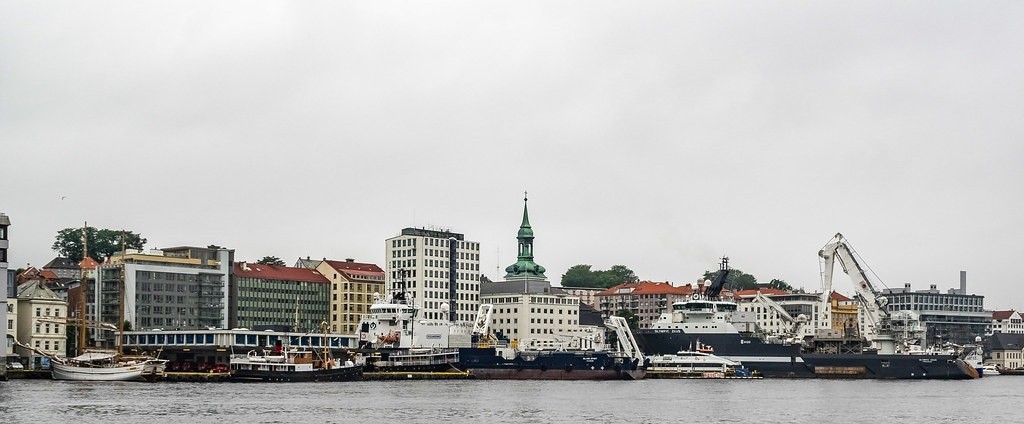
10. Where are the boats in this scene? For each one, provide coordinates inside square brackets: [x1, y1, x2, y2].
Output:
[998, 365, 1024, 376]
[799, 232, 980, 379]
[229, 351, 363, 383]
[630, 293, 826, 376]
[981, 365, 998, 376]
[643, 352, 744, 379]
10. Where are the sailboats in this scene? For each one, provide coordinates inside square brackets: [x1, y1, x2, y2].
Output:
[51, 220, 171, 382]
